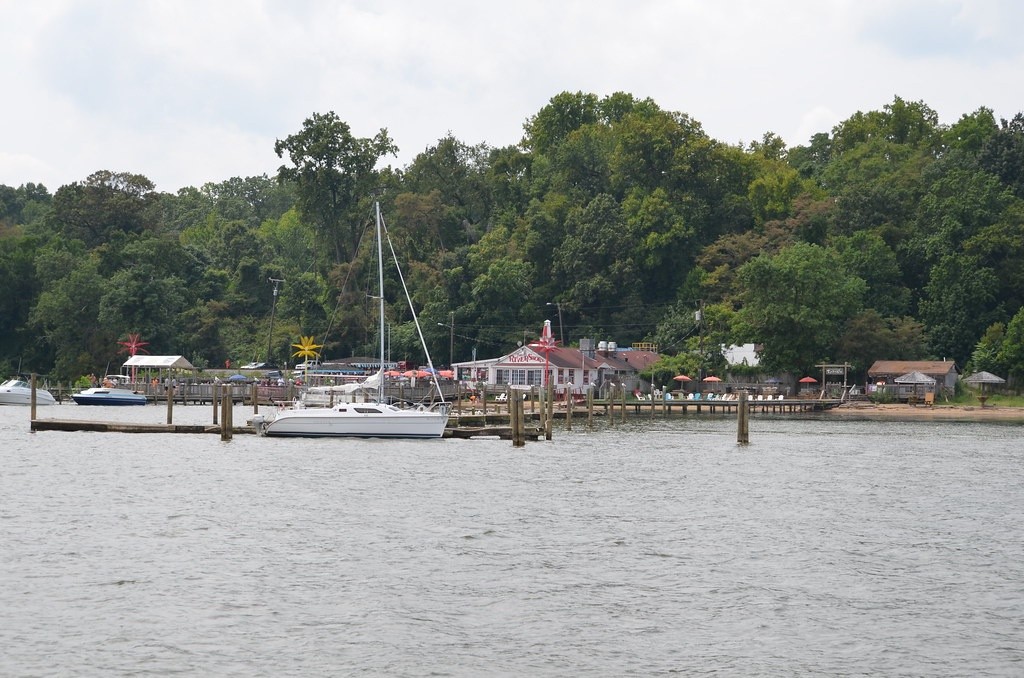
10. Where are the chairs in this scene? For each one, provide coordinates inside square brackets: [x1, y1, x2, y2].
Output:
[924, 392, 933, 406]
[636, 388, 784, 400]
[495, 393, 527, 401]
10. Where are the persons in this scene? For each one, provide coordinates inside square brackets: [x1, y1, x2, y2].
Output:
[86, 371, 111, 387]
[254, 376, 303, 387]
[153, 377, 176, 396]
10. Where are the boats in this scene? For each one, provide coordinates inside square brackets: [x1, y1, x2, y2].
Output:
[0, 358, 57, 406]
[70, 362, 148, 407]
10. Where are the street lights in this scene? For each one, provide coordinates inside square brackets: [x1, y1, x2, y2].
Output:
[545, 302, 564, 346]
[437, 322, 454, 370]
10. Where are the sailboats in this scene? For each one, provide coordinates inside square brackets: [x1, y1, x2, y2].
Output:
[261, 200, 453, 439]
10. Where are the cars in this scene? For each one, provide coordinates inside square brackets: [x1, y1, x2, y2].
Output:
[240, 362, 279, 371]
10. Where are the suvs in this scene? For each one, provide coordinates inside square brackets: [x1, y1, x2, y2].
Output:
[294, 359, 321, 371]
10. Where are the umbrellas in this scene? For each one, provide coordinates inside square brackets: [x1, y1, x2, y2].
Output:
[229, 374, 247, 384]
[383, 368, 455, 378]
[703, 376, 722, 390]
[673, 375, 691, 389]
[894, 370, 936, 395]
[962, 370, 1006, 405]
[799, 376, 818, 393]
[764, 378, 784, 387]
